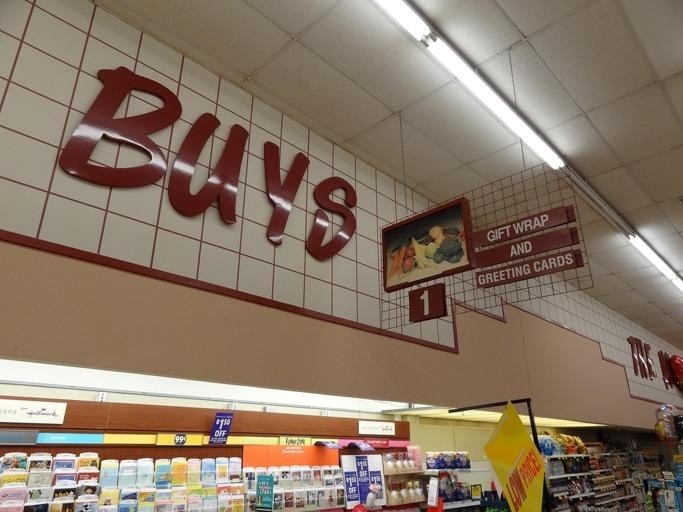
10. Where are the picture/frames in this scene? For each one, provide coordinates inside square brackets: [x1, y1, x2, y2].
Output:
[381, 197, 476, 293]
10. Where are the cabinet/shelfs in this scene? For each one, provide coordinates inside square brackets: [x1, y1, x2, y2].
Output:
[528, 434, 663, 508]
[0, 395, 425, 511]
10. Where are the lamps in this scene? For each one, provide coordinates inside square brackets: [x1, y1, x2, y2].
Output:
[626, 233, 683, 293]
[373, 0, 566, 171]
